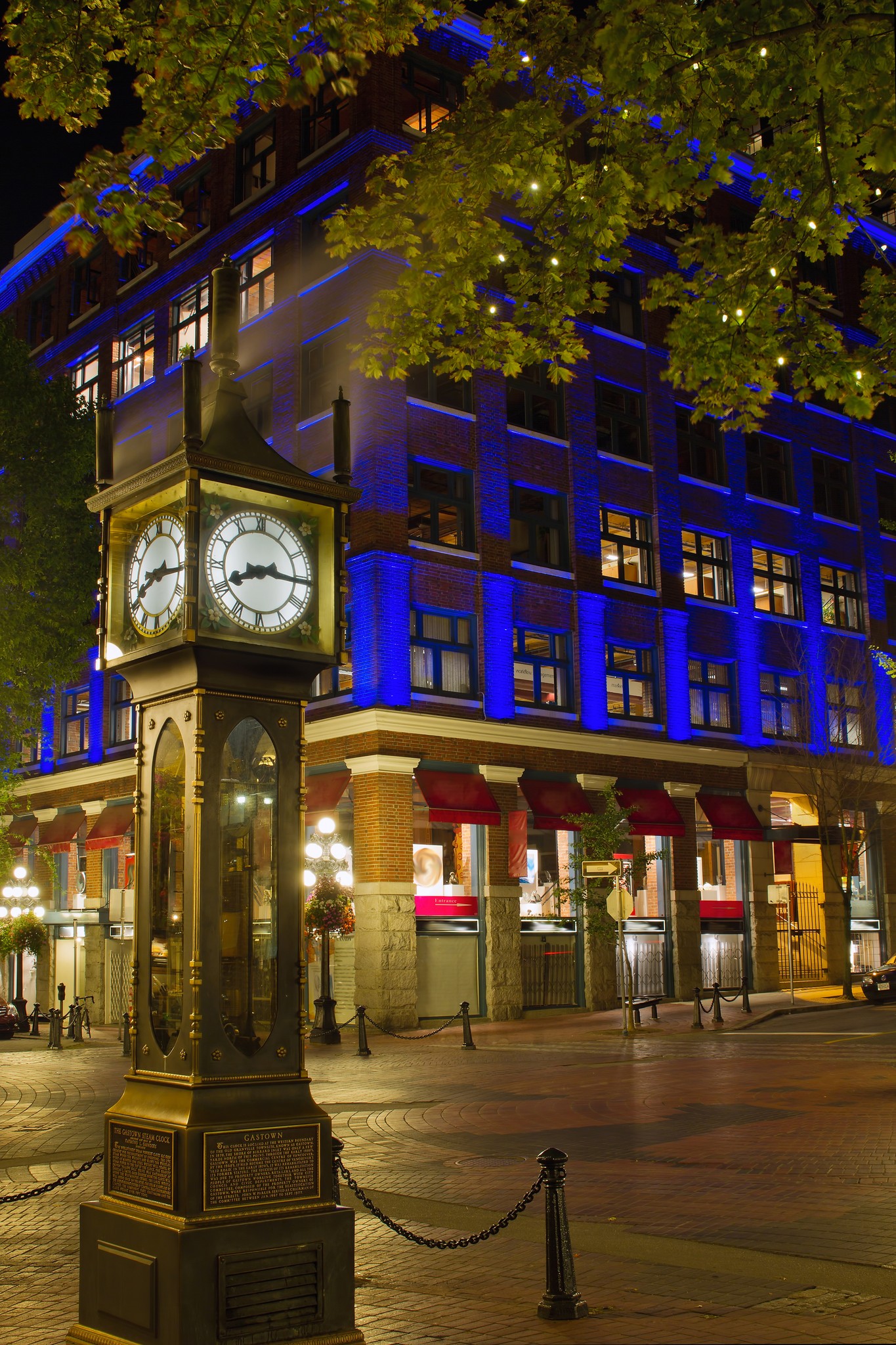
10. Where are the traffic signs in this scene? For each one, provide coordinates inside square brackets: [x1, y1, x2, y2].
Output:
[582, 860, 623, 879]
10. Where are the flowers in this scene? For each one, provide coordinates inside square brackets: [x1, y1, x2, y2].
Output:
[303, 872, 355, 940]
[0, 910, 47, 962]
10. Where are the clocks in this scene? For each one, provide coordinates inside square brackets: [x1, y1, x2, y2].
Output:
[127, 509, 185, 638]
[205, 510, 313, 635]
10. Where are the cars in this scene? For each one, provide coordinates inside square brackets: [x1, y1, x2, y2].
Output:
[861, 952, 896, 1006]
[0, 995, 20, 1040]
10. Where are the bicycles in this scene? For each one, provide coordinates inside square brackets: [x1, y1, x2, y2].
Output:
[73, 995, 96, 1039]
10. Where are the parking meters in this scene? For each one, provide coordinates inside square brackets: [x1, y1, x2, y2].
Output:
[57, 982, 66, 1037]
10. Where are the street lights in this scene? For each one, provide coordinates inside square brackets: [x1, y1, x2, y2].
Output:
[0, 864, 45, 1031]
[303, 818, 354, 1043]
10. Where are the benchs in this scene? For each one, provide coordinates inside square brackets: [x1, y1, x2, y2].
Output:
[625, 995, 667, 1025]
